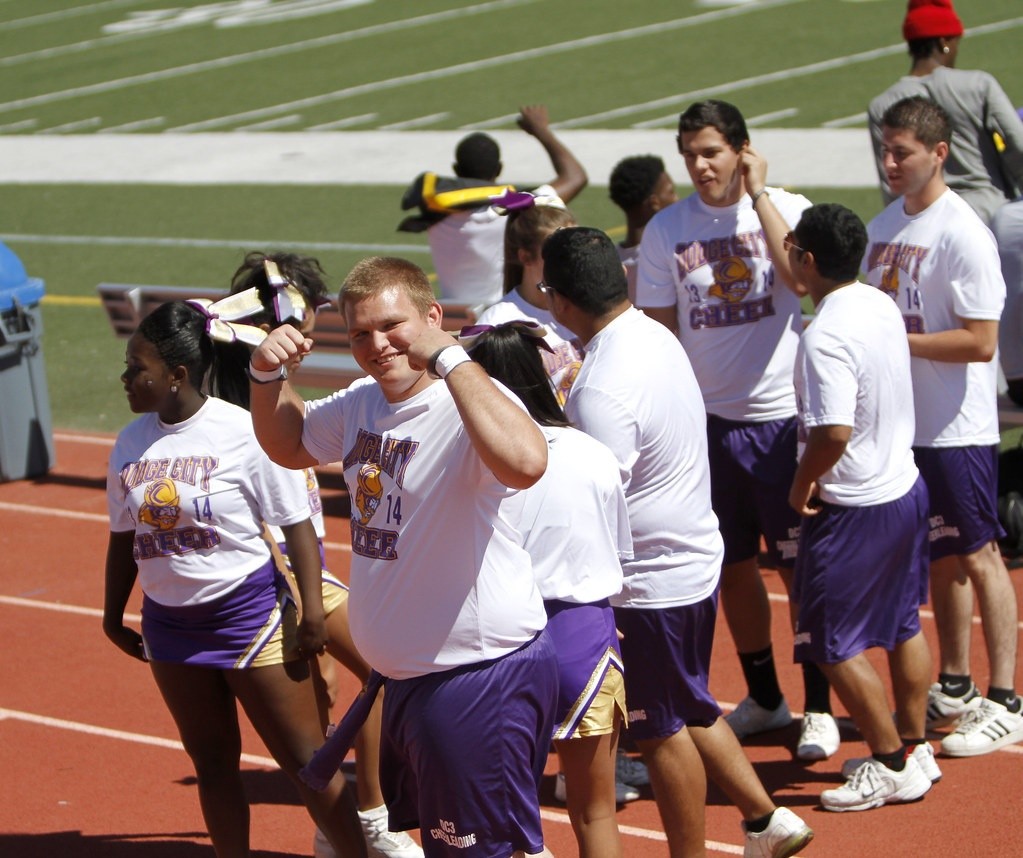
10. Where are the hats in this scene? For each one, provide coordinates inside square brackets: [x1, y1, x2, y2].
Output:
[903, 0, 964, 40]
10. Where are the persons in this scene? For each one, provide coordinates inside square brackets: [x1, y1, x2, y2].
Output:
[102, 242, 561, 858]
[784, 98, 1023, 810]
[866, 0, 1022, 571]
[397, 103, 815, 858]
[608, 150, 680, 298]
[635, 99, 842, 762]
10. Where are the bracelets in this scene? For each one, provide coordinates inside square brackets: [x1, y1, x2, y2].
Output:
[429, 343, 473, 379]
[752, 190, 770, 210]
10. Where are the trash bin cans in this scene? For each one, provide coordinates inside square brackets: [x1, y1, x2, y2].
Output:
[0, 241, 57, 481]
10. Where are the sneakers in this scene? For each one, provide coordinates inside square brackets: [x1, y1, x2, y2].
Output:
[556, 772, 640, 801]
[842, 741, 942, 782]
[314, 826, 336, 858]
[940, 695, 1023, 757]
[797, 711, 841, 759]
[615, 748, 649, 785]
[822, 754, 933, 812]
[741, 807, 814, 858]
[891, 682, 982, 731]
[722, 695, 792, 738]
[358, 811, 425, 858]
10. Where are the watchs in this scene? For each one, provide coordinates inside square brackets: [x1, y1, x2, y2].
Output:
[244, 362, 288, 385]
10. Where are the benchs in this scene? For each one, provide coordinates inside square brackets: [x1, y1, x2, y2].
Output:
[97, 280, 486, 391]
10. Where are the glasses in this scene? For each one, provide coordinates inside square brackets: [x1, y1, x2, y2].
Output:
[782, 232, 806, 251]
[537, 282, 555, 293]
